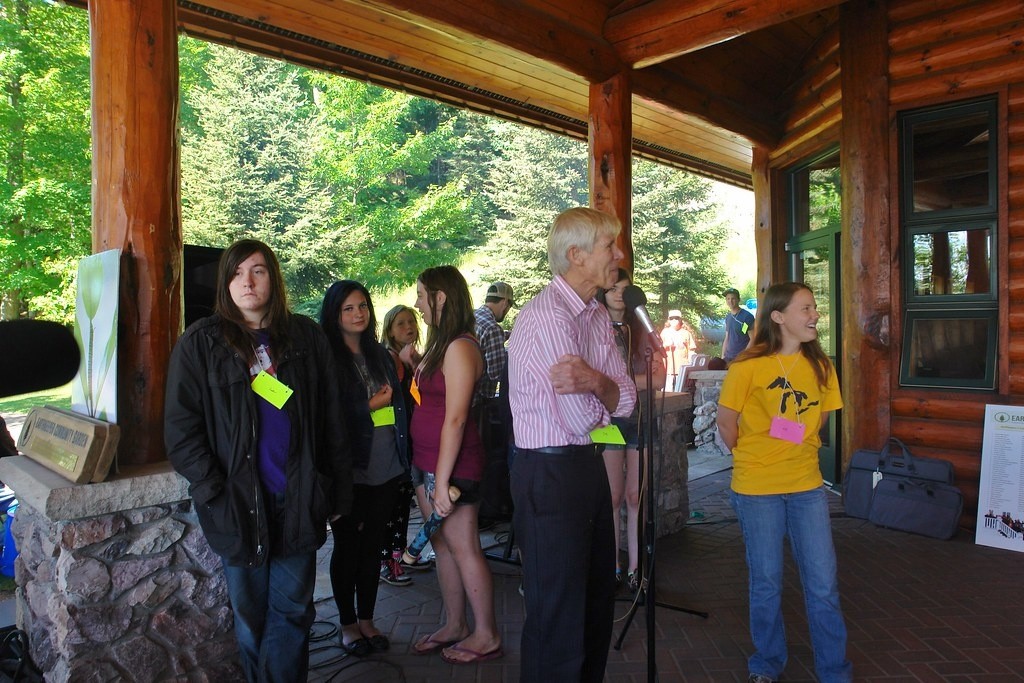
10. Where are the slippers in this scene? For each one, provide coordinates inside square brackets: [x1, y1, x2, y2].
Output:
[441, 641, 502, 666]
[412, 629, 461, 656]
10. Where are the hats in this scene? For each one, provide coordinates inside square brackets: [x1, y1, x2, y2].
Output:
[669, 310, 683, 319]
[487, 280, 517, 309]
[724, 288, 740, 298]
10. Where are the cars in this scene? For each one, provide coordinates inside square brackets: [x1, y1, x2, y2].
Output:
[701, 316, 726, 344]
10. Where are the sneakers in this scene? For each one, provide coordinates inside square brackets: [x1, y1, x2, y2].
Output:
[391, 550, 431, 569]
[379, 559, 412, 587]
[616, 572, 624, 591]
[627, 569, 644, 605]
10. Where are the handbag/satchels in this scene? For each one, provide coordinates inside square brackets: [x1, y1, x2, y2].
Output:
[842, 437, 964, 539]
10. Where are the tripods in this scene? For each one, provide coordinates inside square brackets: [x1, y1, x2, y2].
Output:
[608, 322, 710, 652]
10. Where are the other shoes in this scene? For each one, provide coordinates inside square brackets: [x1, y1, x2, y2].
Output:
[359, 626, 389, 652]
[338, 627, 370, 657]
[478, 517, 498, 531]
[748, 672, 773, 683]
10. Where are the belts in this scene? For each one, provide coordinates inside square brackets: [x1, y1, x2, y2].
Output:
[535, 443, 606, 458]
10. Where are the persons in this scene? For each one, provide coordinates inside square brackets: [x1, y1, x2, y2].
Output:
[380, 306, 434, 584]
[721, 288, 754, 364]
[596, 266, 666, 595]
[164, 239, 373, 683]
[717, 283, 855, 682]
[409, 268, 503, 663]
[510, 212, 637, 683]
[319, 282, 407, 656]
[661, 313, 696, 393]
[473, 283, 517, 531]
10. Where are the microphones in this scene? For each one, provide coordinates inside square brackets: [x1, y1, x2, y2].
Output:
[622, 285, 668, 361]
